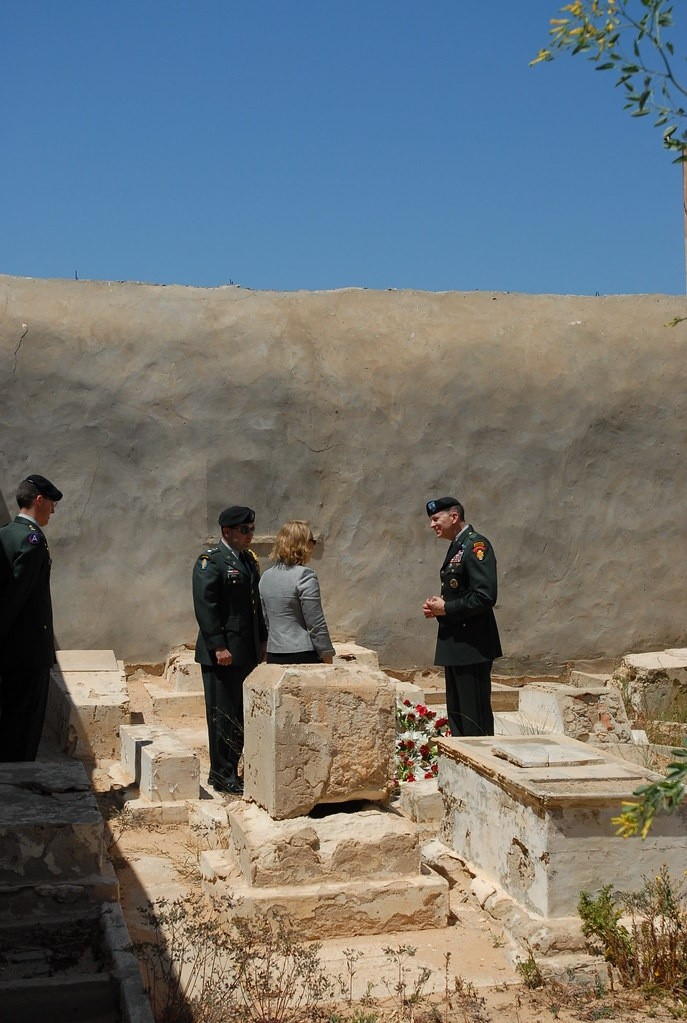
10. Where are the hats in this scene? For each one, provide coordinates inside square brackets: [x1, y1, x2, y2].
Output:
[25, 475, 63, 502]
[426, 497, 461, 517]
[218, 506, 255, 527]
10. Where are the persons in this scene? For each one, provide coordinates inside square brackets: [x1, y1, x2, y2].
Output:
[192, 506, 268, 793]
[422, 496, 504, 737]
[258, 519, 336, 664]
[0, 474, 63, 763]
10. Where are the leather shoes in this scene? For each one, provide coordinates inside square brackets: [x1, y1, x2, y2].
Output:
[208, 776, 244, 796]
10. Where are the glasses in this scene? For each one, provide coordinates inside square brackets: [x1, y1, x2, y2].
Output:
[309, 539, 316, 545]
[54, 501, 58, 506]
[232, 526, 255, 535]
